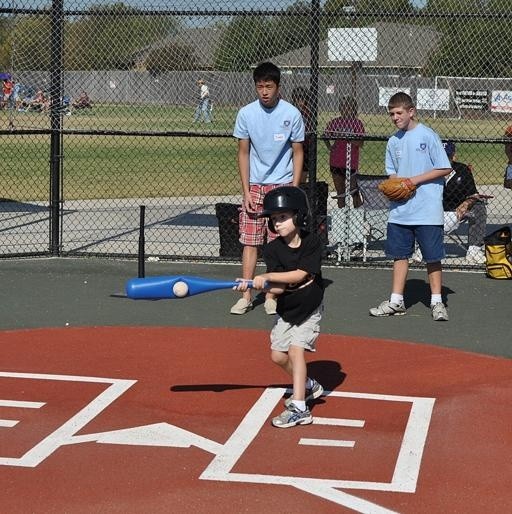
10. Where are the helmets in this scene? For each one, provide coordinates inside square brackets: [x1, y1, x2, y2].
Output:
[255, 185, 312, 234]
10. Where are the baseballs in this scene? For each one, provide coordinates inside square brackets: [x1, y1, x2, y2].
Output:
[172, 281, 188, 296]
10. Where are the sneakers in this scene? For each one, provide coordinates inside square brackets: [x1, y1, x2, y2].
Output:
[284, 377, 325, 407]
[411, 246, 423, 263]
[230, 298, 253, 315]
[430, 301, 449, 321]
[465, 249, 487, 264]
[272, 403, 313, 428]
[369, 300, 407, 317]
[265, 298, 278, 315]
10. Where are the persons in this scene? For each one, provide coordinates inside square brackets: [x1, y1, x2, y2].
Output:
[232, 187, 326, 429]
[229, 63, 306, 316]
[370, 91, 451, 321]
[188, 79, 213, 128]
[441, 140, 491, 265]
[322, 97, 369, 208]
[74, 90, 90, 111]
[291, 85, 316, 184]
[503, 124, 512, 190]
[0, 73, 72, 113]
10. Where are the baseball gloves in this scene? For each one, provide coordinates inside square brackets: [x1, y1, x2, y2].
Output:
[378, 176, 416, 204]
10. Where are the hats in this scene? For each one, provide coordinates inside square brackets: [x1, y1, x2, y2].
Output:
[441, 141, 456, 159]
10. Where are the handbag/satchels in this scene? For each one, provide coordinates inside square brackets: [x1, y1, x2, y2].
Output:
[483, 225, 512, 280]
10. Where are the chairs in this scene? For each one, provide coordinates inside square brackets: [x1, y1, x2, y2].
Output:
[355, 171, 469, 264]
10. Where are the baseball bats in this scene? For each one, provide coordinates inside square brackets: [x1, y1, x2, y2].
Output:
[125, 276, 271, 300]
